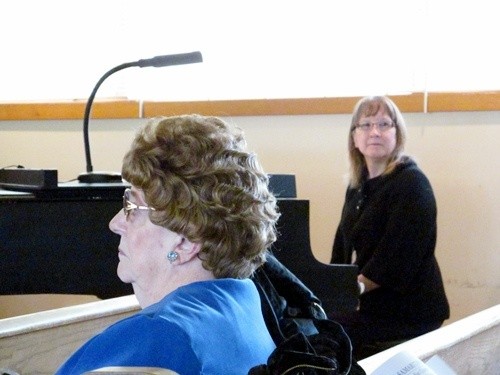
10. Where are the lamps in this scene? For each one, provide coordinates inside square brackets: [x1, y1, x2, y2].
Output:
[78, 52, 203, 183]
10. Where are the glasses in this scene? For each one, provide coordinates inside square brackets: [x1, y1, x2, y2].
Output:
[123, 188, 157, 222]
[351, 119, 396, 131]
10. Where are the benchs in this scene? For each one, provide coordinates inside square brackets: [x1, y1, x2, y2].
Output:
[0, 294, 500, 375]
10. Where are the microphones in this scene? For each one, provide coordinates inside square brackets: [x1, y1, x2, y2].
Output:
[77, 51, 204, 184]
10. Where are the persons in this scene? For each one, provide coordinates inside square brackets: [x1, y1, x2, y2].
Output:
[331, 96, 449, 342]
[58, 115, 278, 374]
